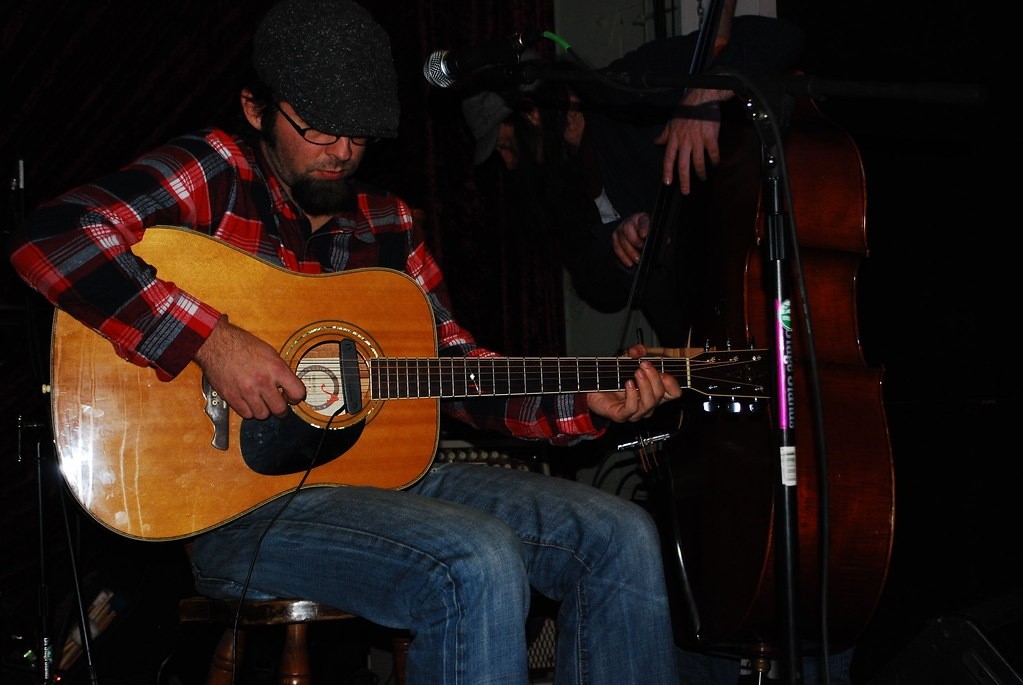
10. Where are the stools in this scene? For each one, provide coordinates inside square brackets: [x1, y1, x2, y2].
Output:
[178, 596, 410, 685]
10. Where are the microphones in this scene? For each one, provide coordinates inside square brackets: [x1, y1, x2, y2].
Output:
[424, 32, 531, 89]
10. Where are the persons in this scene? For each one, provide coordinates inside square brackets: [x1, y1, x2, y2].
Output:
[465, 1, 855, 684]
[7, 1, 682, 685]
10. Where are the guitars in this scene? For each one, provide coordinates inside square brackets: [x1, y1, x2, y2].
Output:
[50, 220, 776, 545]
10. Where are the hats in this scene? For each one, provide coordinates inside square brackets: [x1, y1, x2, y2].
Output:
[252, 0, 400, 138]
[464, 48, 544, 164]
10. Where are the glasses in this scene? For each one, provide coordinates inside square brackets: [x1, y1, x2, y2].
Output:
[265, 96, 379, 146]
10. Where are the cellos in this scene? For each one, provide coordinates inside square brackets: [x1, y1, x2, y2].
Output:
[665, 1, 901, 683]
